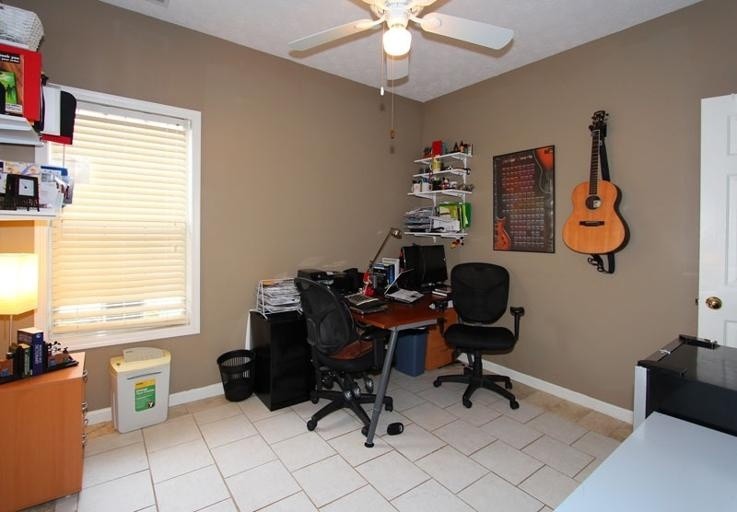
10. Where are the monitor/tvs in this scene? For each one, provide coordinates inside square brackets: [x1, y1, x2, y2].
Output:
[401, 244, 449, 293]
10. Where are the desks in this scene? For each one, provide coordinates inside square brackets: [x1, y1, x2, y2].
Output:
[552, 411, 737, 512]
[352, 287, 458, 448]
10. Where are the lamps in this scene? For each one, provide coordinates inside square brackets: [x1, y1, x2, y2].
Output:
[383, 24, 412, 56]
[0, 252, 39, 346]
[366, 227, 402, 274]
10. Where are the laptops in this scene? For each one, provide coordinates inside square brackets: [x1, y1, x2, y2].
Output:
[384, 268, 424, 303]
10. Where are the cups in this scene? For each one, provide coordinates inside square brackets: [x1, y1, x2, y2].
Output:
[429, 161, 441, 171]
[411, 183, 433, 192]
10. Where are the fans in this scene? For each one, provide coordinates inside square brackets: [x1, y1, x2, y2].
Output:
[287, 0, 515, 81]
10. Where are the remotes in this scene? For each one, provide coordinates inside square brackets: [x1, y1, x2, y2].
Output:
[679, 334, 717, 348]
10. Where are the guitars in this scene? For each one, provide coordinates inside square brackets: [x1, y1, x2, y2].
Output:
[563, 110, 629, 255]
[534, 145, 555, 255]
[493, 156, 512, 251]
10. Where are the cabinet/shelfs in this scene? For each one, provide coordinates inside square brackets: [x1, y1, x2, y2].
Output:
[0, 114, 58, 221]
[0, 352, 87, 512]
[250, 311, 316, 411]
[404, 152, 473, 244]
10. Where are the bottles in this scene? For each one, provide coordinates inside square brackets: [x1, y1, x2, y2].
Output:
[454, 139, 468, 154]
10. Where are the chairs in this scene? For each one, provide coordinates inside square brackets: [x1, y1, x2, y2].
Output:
[431, 263, 524, 409]
[293, 278, 395, 437]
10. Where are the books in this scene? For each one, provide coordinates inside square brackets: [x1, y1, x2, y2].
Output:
[431, 286, 452, 297]
[17, 327, 44, 377]
[372, 257, 401, 291]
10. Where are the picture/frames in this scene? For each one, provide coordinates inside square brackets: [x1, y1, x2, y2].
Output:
[493, 144, 555, 253]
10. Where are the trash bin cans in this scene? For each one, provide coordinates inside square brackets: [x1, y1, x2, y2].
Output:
[217, 349, 256, 402]
[395, 326, 427, 377]
